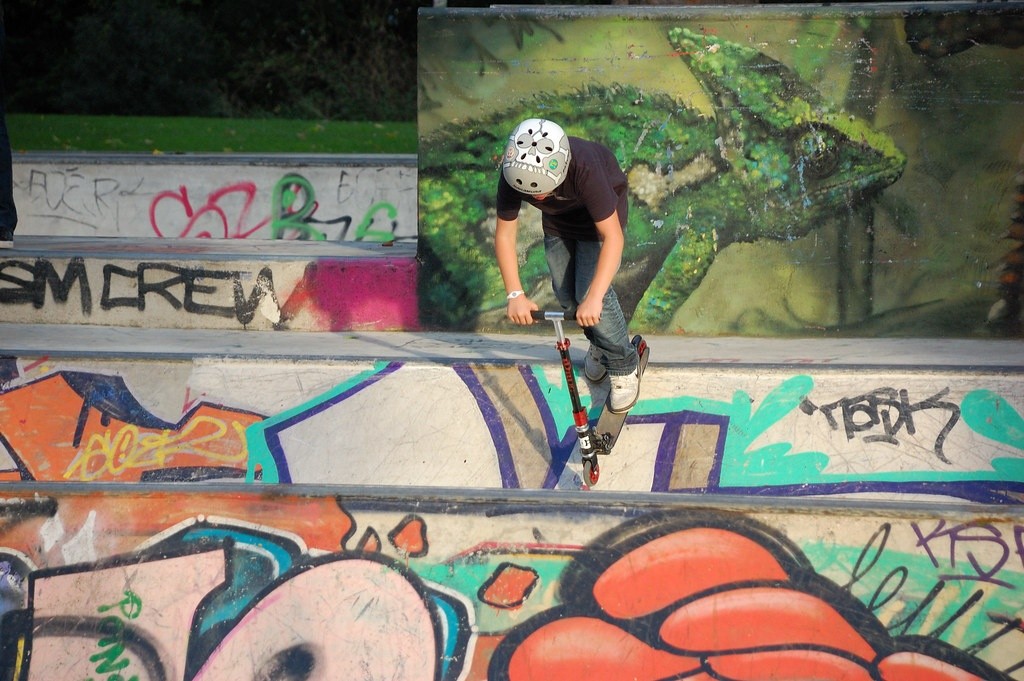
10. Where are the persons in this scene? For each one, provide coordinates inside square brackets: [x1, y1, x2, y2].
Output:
[495, 118, 642, 414]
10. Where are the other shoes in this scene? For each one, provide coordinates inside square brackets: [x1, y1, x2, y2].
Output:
[0, 232, 14, 248]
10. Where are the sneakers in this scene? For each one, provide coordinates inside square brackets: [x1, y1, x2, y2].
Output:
[583, 343, 609, 384]
[609, 352, 640, 414]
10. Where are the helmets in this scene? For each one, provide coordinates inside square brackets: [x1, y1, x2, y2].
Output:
[503, 119, 571, 194]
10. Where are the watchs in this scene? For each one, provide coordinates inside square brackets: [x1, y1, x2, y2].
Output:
[507, 290, 524, 300]
